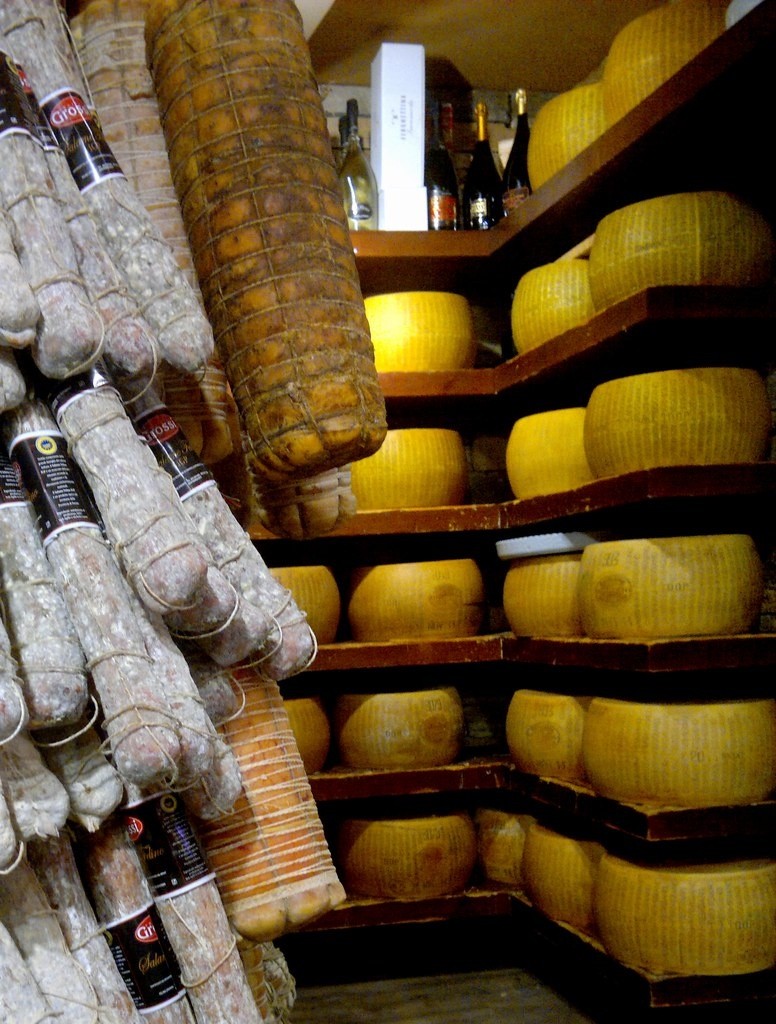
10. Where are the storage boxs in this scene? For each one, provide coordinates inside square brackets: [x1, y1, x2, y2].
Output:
[371, 39, 431, 232]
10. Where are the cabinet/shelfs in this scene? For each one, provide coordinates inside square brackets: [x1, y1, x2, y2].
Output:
[238, 0, 776, 1024]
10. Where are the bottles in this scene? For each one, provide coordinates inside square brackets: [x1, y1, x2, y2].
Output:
[463, 104, 505, 230]
[424, 99, 460, 231]
[337, 98, 379, 233]
[504, 88, 533, 217]
